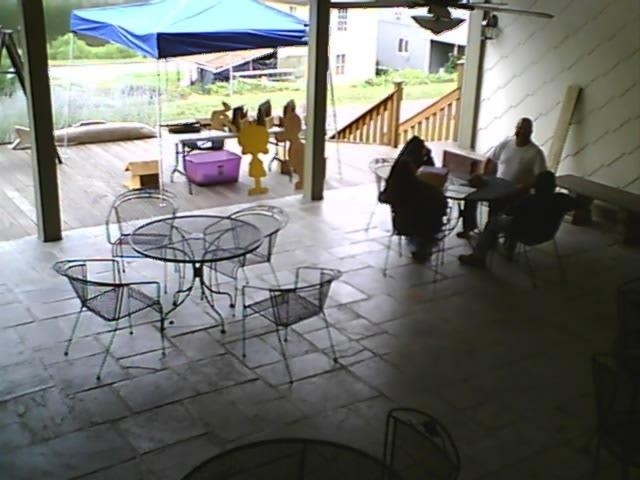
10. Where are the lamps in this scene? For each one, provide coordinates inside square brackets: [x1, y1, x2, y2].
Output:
[409, 6, 465, 32]
[480, 15, 501, 41]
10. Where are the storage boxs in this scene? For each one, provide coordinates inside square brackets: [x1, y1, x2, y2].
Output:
[125, 159, 163, 193]
[183, 150, 242, 183]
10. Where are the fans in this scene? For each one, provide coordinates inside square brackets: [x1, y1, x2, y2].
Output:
[323, 1, 555, 20]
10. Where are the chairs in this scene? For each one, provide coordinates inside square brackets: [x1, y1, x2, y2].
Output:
[380, 407, 460, 480]
[364, 153, 576, 294]
[202, 204, 290, 310]
[105, 189, 178, 295]
[616, 276, 639, 356]
[52, 258, 165, 383]
[241, 266, 341, 386]
[588, 354, 639, 479]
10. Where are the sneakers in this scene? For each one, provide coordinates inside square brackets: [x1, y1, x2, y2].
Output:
[457, 226, 477, 237]
[458, 254, 486, 268]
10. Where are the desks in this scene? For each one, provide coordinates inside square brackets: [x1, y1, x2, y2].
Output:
[130, 214, 266, 335]
[180, 436, 403, 480]
[156, 125, 294, 192]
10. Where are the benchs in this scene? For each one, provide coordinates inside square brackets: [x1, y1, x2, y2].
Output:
[555, 174, 639, 243]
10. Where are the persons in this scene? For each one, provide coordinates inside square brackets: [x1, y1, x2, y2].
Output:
[379, 117, 556, 269]
